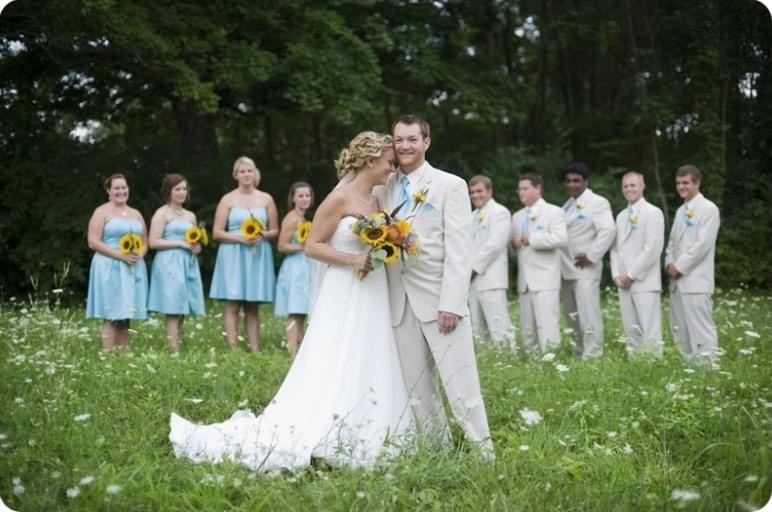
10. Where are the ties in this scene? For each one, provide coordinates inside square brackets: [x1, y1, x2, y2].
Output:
[680, 208, 689, 227]
[476, 211, 481, 227]
[627, 207, 635, 231]
[393, 177, 412, 221]
[520, 206, 534, 238]
[566, 200, 577, 217]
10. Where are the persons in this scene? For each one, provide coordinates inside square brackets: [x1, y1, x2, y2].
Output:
[609, 171, 664, 361]
[665, 164, 721, 364]
[560, 166, 617, 360]
[374, 116, 498, 465]
[509, 173, 569, 353]
[88, 173, 149, 349]
[148, 173, 207, 347]
[210, 157, 279, 352]
[275, 181, 314, 361]
[168, 132, 418, 475]
[467, 175, 513, 345]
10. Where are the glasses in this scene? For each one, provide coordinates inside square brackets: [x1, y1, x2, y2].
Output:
[517, 187, 533, 193]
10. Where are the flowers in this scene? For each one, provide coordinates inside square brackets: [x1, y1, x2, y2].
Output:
[118, 234, 143, 274]
[186, 222, 208, 263]
[241, 212, 264, 240]
[297, 221, 312, 243]
[350, 210, 421, 280]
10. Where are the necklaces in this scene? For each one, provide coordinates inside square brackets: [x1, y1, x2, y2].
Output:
[108, 202, 131, 217]
[169, 204, 188, 217]
[347, 180, 374, 206]
